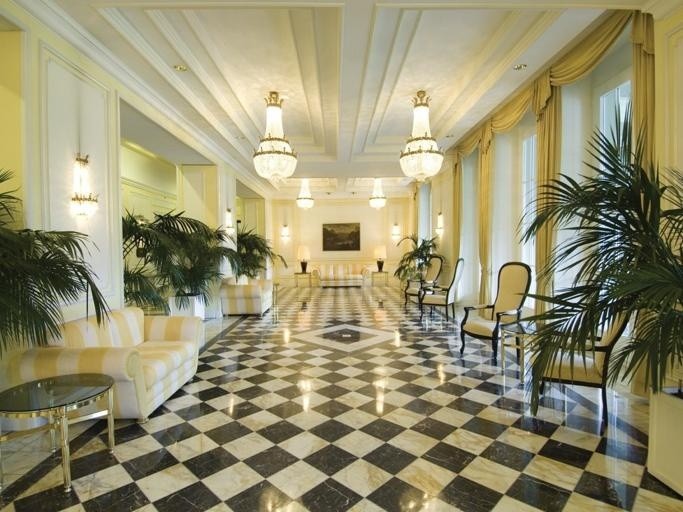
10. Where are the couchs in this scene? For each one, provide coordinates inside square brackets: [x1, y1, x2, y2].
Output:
[1, 305, 203, 424]
[221, 274, 274, 317]
[312, 263, 371, 288]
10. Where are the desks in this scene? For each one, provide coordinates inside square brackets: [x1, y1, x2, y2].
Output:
[294, 272, 311, 287]
[372, 271, 389, 287]
[0, 373, 116, 493]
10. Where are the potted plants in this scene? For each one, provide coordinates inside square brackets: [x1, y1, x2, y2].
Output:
[134, 203, 251, 321]
[501, 98, 683, 502]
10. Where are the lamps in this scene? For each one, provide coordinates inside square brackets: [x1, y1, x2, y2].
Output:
[391, 221, 402, 243]
[71, 151, 100, 217]
[253, 91, 298, 180]
[280, 223, 291, 243]
[368, 179, 388, 211]
[298, 245, 310, 273]
[296, 178, 315, 207]
[399, 90, 444, 182]
[375, 245, 386, 271]
[435, 212, 445, 235]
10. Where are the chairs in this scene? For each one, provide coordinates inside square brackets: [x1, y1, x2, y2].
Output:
[418, 257, 466, 322]
[404, 254, 443, 313]
[531, 294, 636, 427]
[458, 261, 533, 365]
